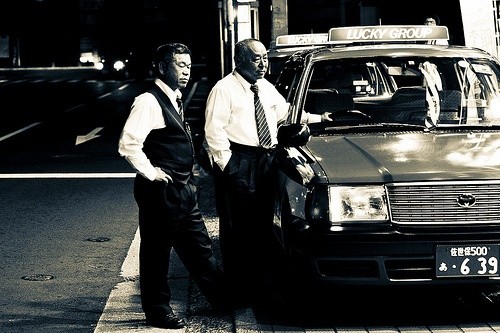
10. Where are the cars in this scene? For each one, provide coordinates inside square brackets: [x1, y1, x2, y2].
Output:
[265, 21, 500, 295]
[95, 50, 156, 78]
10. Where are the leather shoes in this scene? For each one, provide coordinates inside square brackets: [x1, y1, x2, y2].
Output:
[146, 313, 184, 328]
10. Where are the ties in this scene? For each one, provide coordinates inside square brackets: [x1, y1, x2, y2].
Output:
[176, 98, 185, 123]
[250, 86, 272, 149]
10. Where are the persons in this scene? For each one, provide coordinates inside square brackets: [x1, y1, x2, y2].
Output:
[116, 42, 256, 329]
[204, 41, 333, 309]
[423, 18, 449, 46]
